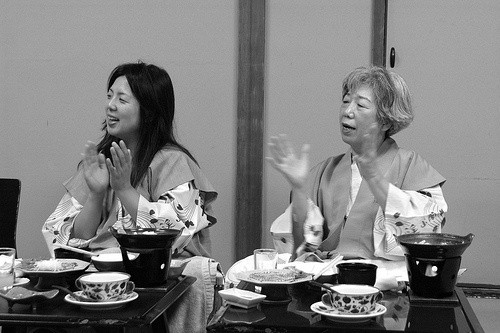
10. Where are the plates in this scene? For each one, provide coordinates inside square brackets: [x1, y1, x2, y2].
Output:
[64, 290, 139, 311]
[218, 287, 266, 309]
[0, 287, 59, 311]
[310, 301, 387, 323]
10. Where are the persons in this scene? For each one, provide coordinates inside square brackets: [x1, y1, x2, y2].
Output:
[225, 67, 448, 292]
[42, 64, 223, 333]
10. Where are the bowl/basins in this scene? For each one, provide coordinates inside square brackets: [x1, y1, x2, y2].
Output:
[320, 284, 384, 314]
[297, 262, 339, 285]
[91, 252, 140, 271]
[336, 263, 378, 286]
[14, 278, 30, 288]
[169, 259, 191, 279]
[79, 271, 131, 302]
[54, 246, 91, 261]
[16, 258, 93, 285]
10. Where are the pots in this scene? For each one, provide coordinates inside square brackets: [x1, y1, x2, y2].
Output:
[108, 225, 185, 249]
[393, 232, 476, 259]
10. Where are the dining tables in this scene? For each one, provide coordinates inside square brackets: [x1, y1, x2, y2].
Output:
[0, 271, 197, 333]
[206, 280, 485, 333]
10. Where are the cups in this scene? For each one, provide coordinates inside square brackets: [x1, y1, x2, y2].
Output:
[0, 247, 15, 291]
[254, 249, 277, 270]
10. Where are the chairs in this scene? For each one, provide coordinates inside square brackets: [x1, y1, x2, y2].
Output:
[0, 178, 21, 259]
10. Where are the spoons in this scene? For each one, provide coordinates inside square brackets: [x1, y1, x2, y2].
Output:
[51, 284, 97, 302]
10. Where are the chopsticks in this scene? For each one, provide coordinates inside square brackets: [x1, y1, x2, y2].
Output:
[313, 254, 344, 281]
[53, 242, 101, 257]
